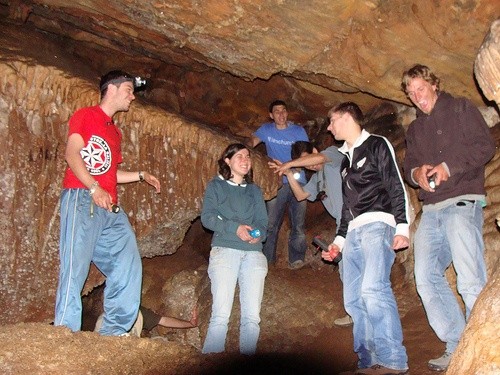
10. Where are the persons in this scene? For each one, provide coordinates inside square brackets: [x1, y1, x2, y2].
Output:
[402, 63, 496, 371]
[54, 70, 160, 337]
[267, 140, 354, 327]
[245, 100, 310, 268]
[201, 143, 268, 353]
[81, 282, 198, 332]
[321, 100, 410, 375]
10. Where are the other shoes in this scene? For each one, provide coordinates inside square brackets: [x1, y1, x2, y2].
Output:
[354, 364, 408, 375]
[427, 352, 452, 371]
[287, 260, 311, 271]
[334, 315, 354, 326]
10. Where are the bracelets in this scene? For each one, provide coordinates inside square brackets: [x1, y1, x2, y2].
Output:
[88, 181, 99, 197]
[138, 170, 144, 182]
